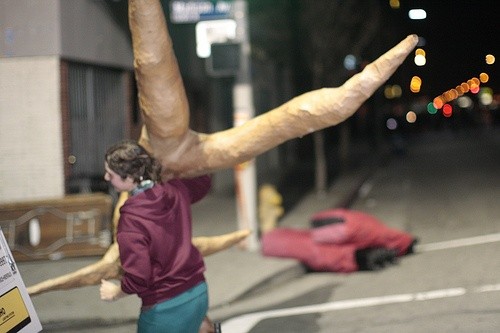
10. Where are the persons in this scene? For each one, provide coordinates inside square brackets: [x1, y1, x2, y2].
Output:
[100, 137, 209, 332]
